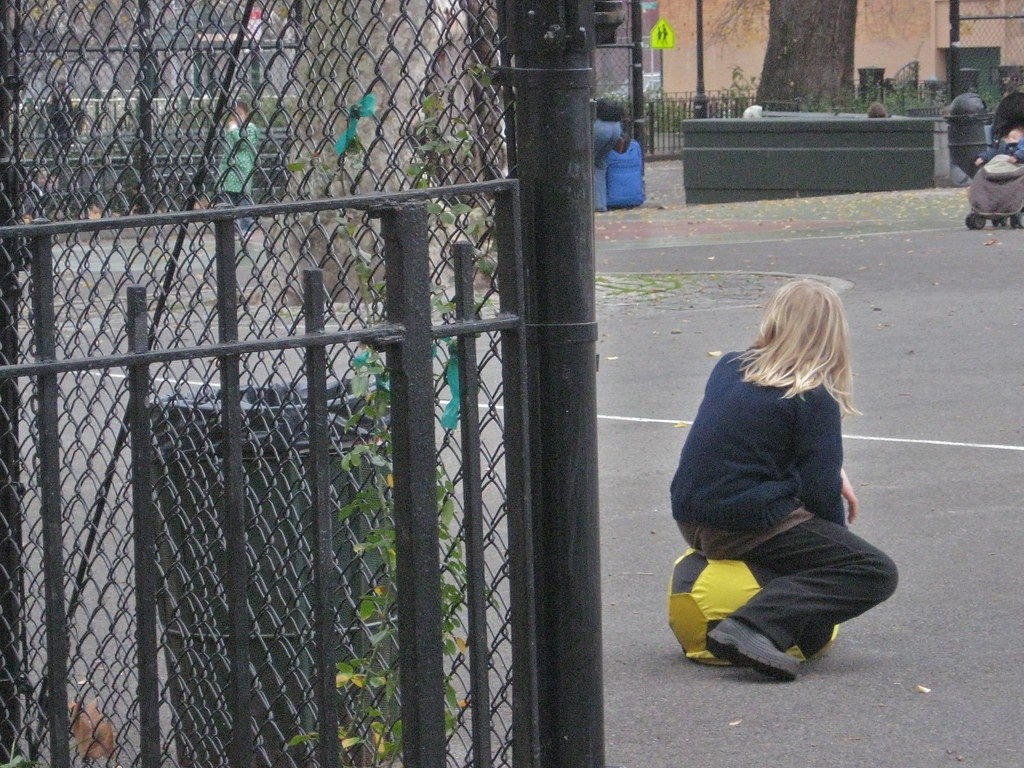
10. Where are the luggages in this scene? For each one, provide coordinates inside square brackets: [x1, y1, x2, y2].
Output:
[607, 140, 646, 207]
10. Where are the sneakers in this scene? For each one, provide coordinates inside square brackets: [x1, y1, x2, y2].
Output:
[707, 617, 799, 680]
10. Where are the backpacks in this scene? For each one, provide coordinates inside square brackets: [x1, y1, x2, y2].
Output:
[594, 98, 624, 121]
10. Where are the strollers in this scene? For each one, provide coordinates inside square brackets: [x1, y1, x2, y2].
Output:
[966, 92, 1024, 230]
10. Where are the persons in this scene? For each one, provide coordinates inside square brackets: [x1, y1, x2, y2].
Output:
[670, 280, 899, 681]
[974, 127, 1024, 166]
[593, 97, 644, 212]
[214, 102, 257, 241]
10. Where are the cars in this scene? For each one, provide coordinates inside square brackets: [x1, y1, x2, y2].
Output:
[621, 73, 660, 98]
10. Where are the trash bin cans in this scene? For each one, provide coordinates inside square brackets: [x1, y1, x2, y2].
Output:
[139, 379, 395, 768]
[945, 92, 997, 188]
